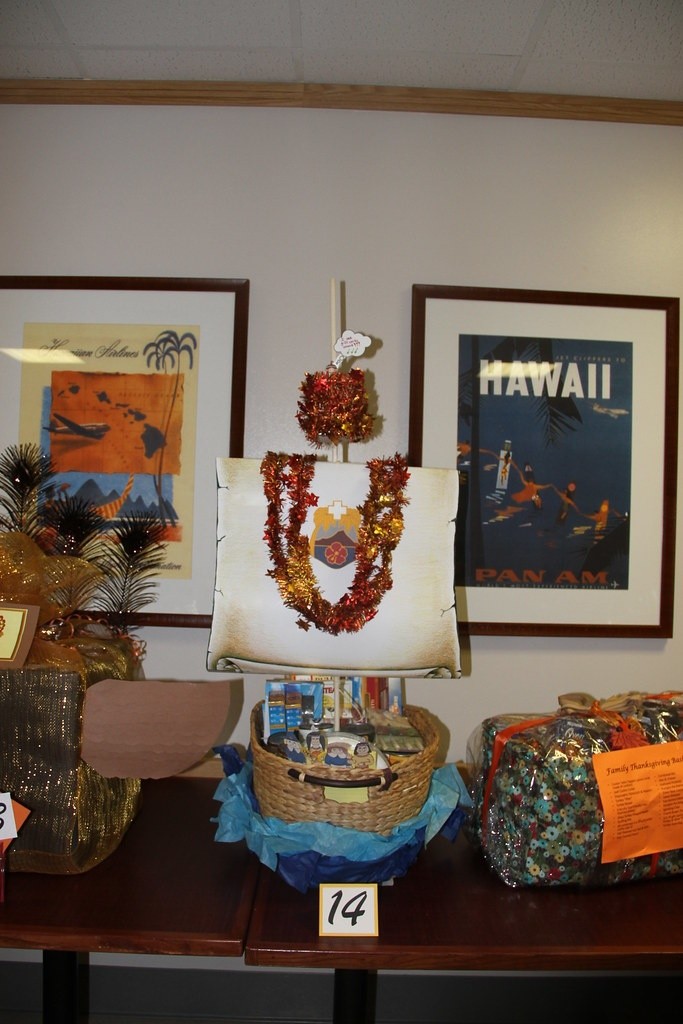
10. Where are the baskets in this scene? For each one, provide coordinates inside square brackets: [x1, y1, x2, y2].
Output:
[250, 701, 439, 838]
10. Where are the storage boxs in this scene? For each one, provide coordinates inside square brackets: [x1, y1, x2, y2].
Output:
[264, 675, 401, 744]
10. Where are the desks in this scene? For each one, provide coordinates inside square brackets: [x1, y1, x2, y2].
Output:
[0, 777, 683, 1024]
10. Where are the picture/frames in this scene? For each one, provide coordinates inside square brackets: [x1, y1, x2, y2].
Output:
[409, 284, 679, 638]
[0, 277, 250, 628]
